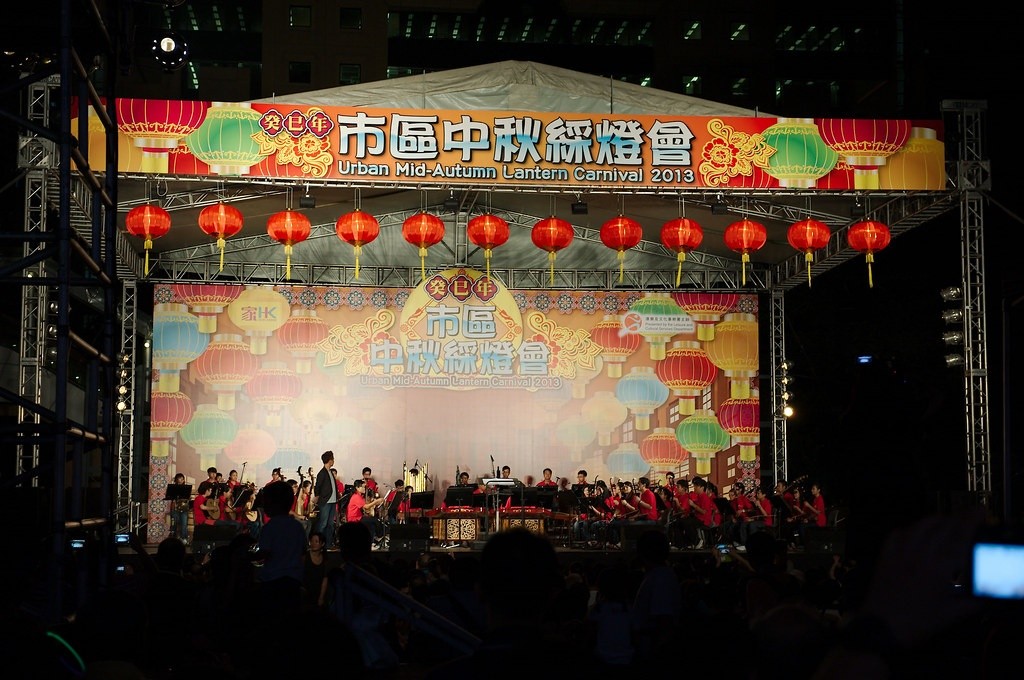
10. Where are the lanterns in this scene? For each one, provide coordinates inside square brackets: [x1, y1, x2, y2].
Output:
[722, 218, 767, 286]
[145, 281, 763, 475]
[752, 117, 945, 190]
[600, 215, 643, 282]
[661, 216, 703, 288]
[335, 209, 380, 280]
[847, 216, 890, 288]
[467, 212, 510, 284]
[402, 212, 445, 281]
[531, 216, 574, 284]
[184, 102, 274, 174]
[266, 209, 311, 280]
[198, 202, 245, 273]
[787, 218, 831, 288]
[115, 99, 207, 178]
[125, 205, 172, 274]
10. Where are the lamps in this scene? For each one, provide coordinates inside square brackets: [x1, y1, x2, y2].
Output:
[115, 369, 126, 378]
[152, 30, 190, 72]
[850, 198, 865, 219]
[115, 402, 126, 411]
[943, 330, 965, 368]
[47, 301, 72, 316]
[444, 191, 461, 212]
[47, 324, 57, 340]
[117, 353, 128, 363]
[939, 285, 964, 303]
[781, 360, 794, 371]
[299, 194, 316, 208]
[711, 196, 728, 215]
[116, 385, 127, 395]
[45, 346, 57, 359]
[781, 391, 794, 401]
[941, 308, 964, 327]
[149, 185, 167, 200]
[781, 374, 795, 385]
[570, 198, 588, 216]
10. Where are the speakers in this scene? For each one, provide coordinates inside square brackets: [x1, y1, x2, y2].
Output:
[621, 523, 663, 551]
[191, 523, 237, 553]
[389, 525, 431, 553]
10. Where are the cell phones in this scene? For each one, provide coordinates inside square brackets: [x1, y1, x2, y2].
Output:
[717, 545, 731, 553]
[114, 533, 130, 544]
[71, 540, 85, 548]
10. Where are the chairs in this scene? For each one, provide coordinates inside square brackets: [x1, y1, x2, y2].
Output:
[574, 505, 846, 550]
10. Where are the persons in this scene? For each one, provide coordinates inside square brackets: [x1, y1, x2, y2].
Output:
[170, 473, 190, 546]
[502, 466, 510, 479]
[536, 468, 557, 487]
[314, 452, 340, 550]
[200, 466, 414, 550]
[554, 470, 829, 552]
[459, 472, 469, 487]
[193, 481, 220, 561]
[1, 522, 1024, 680]
[263, 482, 306, 608]
